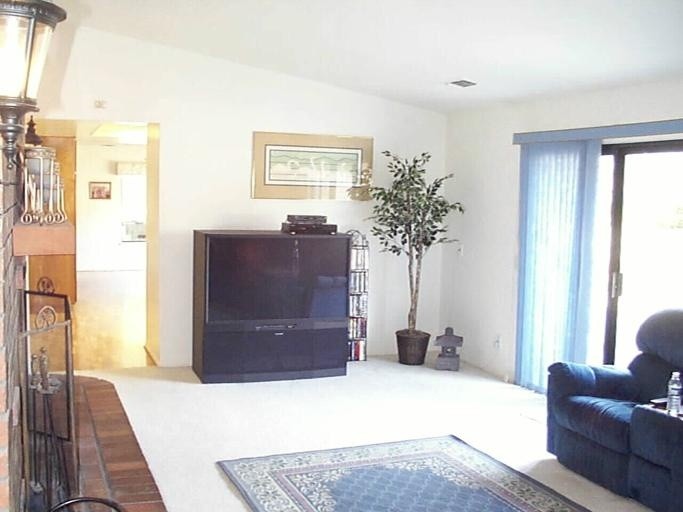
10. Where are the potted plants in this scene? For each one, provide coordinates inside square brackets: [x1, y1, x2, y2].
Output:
[363, 148, 464, 365]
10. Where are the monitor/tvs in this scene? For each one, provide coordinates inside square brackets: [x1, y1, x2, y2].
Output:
[202, 232, 350, 326]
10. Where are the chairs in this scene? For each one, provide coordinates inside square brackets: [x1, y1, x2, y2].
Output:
[545, 307, 683, 510]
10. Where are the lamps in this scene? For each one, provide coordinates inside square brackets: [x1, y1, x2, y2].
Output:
[0, 1, 67, 170]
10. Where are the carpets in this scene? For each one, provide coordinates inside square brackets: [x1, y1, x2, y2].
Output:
[216, 432, 591, 511]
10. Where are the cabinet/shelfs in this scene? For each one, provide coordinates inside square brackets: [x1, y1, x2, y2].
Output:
[193, 230, 351, 383]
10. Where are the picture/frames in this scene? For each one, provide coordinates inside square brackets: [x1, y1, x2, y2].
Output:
[87, 181, 112, 200]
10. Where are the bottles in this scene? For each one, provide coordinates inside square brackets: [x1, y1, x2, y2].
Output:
[666, 371, 683, 418]
